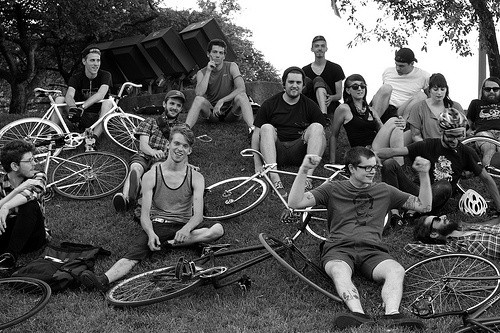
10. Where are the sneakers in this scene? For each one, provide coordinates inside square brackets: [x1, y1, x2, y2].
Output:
[306, 179, 313, 191]
[274, 181, 287, 198]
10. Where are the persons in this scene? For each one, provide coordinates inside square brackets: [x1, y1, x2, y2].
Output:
[251, 63, 328, 199]
[47, 46, 113, 152]
[182, 38, 255, 143]
[79, 124, 225, 292]
[287, 147, 433, 332]
[369, 50, 430, 125]
[110, 90, 186, 210]
[329, 72, 500, 259]
[301, 35, 346, 127]
[0, 140, 52, 268]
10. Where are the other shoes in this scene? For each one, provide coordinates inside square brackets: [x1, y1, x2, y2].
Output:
[334, 313, 374, 329]
[382, 214, 403, 237]
[379, 313, 424, 330]
[128, 169, 139, 201]
[247, 127, 254, 144]
[80, 270, 108, 294]
[85, 136, 97, 153]
[402, 212, 415, 228]
[112, 193, 126, 213]
[157, 240, 173, 254]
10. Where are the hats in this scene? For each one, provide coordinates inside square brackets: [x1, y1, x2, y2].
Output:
[313, 36, 325, 41]
[395, 48, 418, 63]
[164, 90, 186, 104]
[283, 66, 305, 84]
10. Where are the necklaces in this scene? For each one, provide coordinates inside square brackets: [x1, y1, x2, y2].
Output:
[355, 104, 366, 116]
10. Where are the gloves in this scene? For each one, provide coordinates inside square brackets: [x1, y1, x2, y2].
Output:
[66, 106, 84, 123]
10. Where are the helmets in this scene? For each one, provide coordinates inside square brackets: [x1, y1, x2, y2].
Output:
[438, 107, 466, 131]
[459, 190, 488, 217]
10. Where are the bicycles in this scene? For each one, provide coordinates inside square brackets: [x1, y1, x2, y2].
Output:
[0, 80, 147, 166]
[203, 149, 393, 243]
[0, 134, 130, 201]
[401, 251, 500, 333]
[0, 251, 53, 330]
[457, 134, 500, 203]
[108, 222, 345, 303]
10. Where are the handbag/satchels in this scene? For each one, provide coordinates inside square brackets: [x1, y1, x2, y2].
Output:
[8, 238, 111, 295]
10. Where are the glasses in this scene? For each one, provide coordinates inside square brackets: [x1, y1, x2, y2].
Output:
[354, 165, 379, 172]
[430, 215, 442, 233]
[15, 157, 33, 164]
[348, 83, 366, 90]
[444, 131, 464, 138]
[482, 87, 500, 92]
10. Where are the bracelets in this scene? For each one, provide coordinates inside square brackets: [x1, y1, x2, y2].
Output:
[329, 161, 335, 164]
